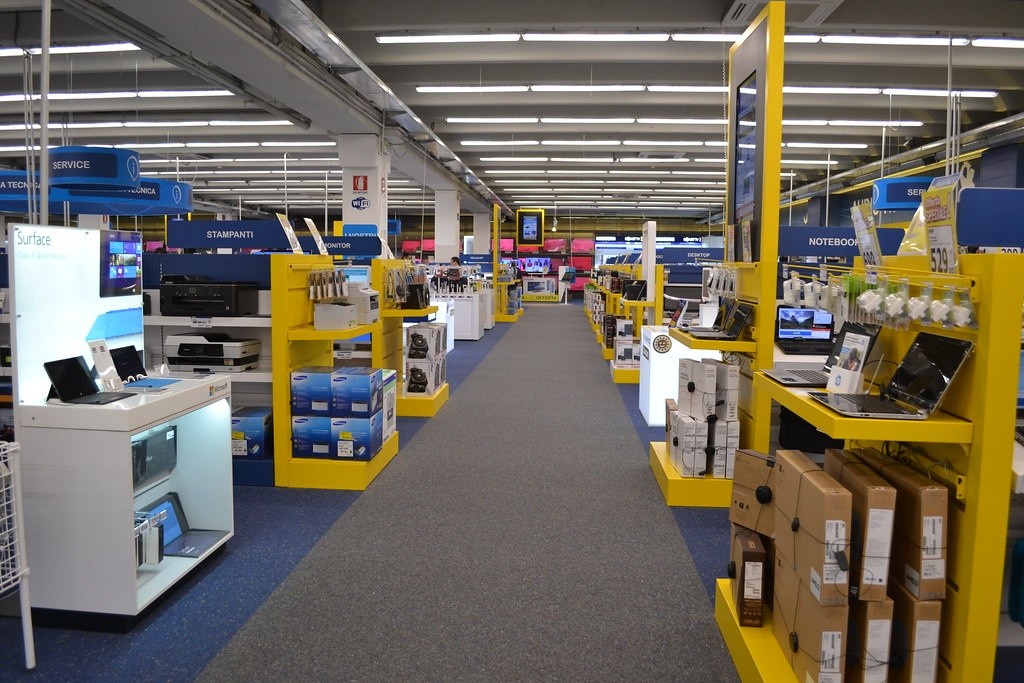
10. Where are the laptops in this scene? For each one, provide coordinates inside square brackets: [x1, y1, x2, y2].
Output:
[759, 320, 881, 386]
[141, 494, 230, 558]
[108, 345, 182, 389]
[43, 355, 138, 405]
[775, 305, 835, 355]
[678, 296, 753, 340]
[808, 331, 973, 420]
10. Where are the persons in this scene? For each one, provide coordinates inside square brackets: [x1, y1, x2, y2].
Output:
[402, 252, 412, 260]
[508, 261, 522, 296]
[450, 257, 461, 266]
[526, 259, 548, 266]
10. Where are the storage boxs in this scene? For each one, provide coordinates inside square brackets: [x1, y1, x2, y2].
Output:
[290, 366, 397, 460]
[665, 359, 740, 480]
[516, 286, 522, 309]
[729, 449, 948, 683]
[231, 406, 274, 460]
[508, 290, 518, 315]
[405, 321, 447, 396]
[591, 291, 641, 368]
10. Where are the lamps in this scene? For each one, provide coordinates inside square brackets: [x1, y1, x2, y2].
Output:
[551, 204, 558, 232]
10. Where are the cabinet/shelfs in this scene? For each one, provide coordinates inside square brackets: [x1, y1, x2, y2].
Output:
[609, 264, 664, 384]
[493, 279, 524, 322]
[371, 259, 449, 418]
[142, 254, 275, 487]
[20, 371, 234, 633]
[715, 373, 1024, 683]
[583, 263, 642, 360]
[270, 254, 399, 490]
[648, 324, 825, 508]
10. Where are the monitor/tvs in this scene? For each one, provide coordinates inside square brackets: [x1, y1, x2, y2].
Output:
[664, 286, 702, 312]
